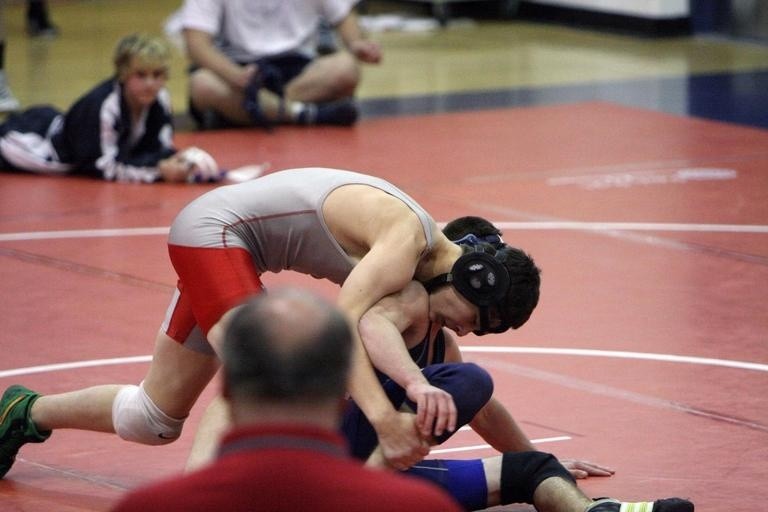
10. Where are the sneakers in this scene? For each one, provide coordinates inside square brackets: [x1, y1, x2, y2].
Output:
[317, 99, 360, 127]
[0, 385, 55, 480]
[585, 496, 695, 512]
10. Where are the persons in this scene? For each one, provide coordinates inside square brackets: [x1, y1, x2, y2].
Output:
[0, 166, 539, 478]
[167, 1, 382, 131]
[115, 287, 459, 512]
[0, 33, 228, 187]
[185, 217, 695, 512]
[23, 0, 59, 38]
